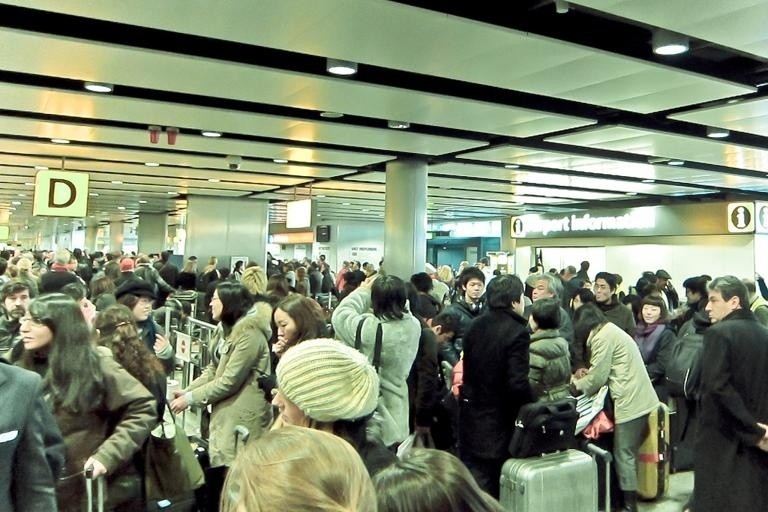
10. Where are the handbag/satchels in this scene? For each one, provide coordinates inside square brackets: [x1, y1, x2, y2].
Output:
[132, 423, 206, 511]
[424, 382, 458, 449]
[509, 399, 580, 458]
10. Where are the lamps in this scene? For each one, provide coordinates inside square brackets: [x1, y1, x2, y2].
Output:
[83, 81, 114, 93]
[650, 27, 690, 57]
[326, 58, 358, 76]
[706, 124, 729, 138]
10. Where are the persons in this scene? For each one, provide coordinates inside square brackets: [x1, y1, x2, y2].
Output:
[1, 239, 767, 510]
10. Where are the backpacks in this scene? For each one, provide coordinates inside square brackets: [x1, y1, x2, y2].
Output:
[663, 319, 705, 398]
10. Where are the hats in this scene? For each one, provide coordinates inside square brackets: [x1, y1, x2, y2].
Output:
[115, 280, 158, 300]
[276, 339, 379, 421]
[120, 258, 135, 273]
[656, 269, 672, 279]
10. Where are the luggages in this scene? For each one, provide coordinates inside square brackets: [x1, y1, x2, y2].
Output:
[637, 401, 670, 500]
[499, 450, 599, 511]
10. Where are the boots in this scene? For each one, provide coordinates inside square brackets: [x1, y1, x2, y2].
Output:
[620, 491, 637, 512]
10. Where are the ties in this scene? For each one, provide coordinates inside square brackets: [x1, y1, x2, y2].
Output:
[473, 302, 481, 312]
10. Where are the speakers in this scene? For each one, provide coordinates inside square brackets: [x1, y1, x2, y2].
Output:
[316, 224, 330, 242]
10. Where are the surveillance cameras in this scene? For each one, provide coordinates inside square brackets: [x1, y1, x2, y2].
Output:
[225, 156, 241, 169]
